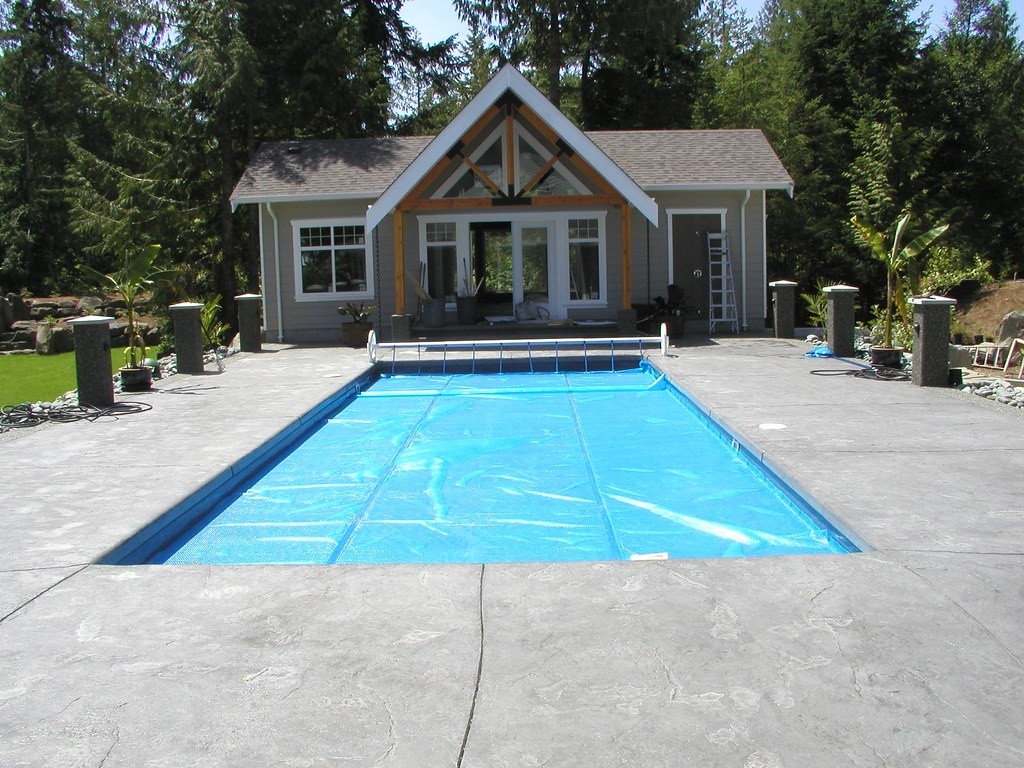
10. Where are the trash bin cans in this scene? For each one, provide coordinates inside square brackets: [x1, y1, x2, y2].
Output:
[457, 296, 478, 326]
[423, 298, 445, 328]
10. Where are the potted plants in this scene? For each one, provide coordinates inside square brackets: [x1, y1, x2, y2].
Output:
[849, 213, 949, 368]
[74, 245, 182, 390]
[337, 301, 378, 346]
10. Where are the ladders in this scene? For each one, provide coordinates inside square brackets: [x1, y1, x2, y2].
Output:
[706, 229, 740, 337]
